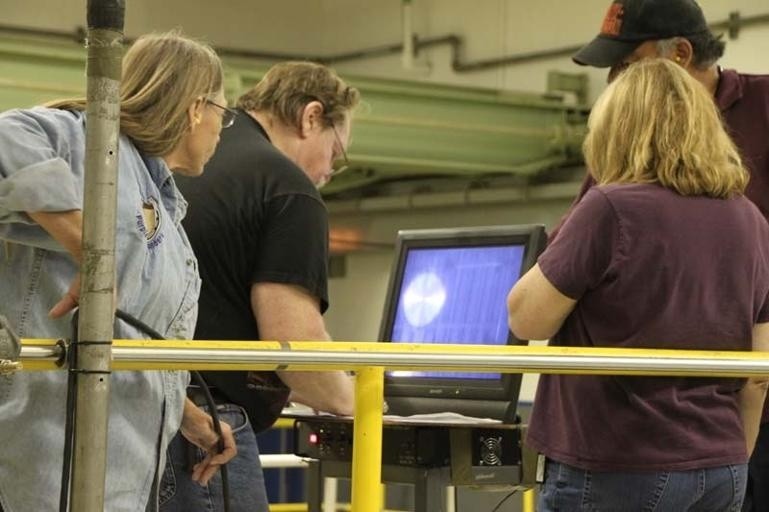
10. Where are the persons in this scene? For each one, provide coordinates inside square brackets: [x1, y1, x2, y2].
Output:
[567, 2, 769, 512]
[504, 56, 769, 511]
[1, 31, 237, 509]
[157, 59, 390, 512]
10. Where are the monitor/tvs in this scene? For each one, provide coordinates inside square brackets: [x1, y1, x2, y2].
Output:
[377, 223, 547, 425]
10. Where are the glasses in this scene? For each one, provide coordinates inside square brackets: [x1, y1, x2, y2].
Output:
[204, 98, 238, 128]
[328, 120, 350, 176]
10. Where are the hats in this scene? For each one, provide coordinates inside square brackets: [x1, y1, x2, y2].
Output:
[573, 0, 705, 66]
